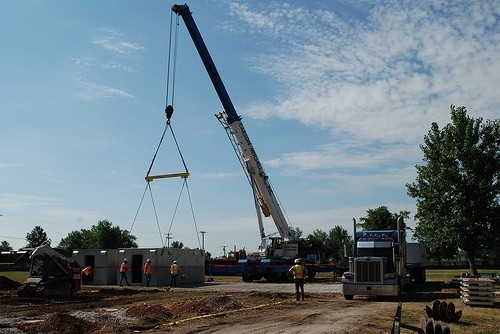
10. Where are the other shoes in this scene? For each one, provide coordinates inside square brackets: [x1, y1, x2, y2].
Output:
[146, 285, 151, 287]
[170, 285, 172, 287]
[173, 286, 177, 287]
[301, 298, 304, 301]
[120, 284, 124, 286]
[294, 298, 300, 301]
[128, 284, 132, 286]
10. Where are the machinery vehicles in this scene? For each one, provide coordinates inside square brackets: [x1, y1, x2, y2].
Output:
[341, 215, 430, 300]
[16, 244, 84, 299]
[144, 2, 337, 283]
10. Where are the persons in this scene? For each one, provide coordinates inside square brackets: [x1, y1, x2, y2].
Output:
[81, 265, 94, 285]
[170, 261, 180, 287]
[289, 259, 308, 301]
[144, 259, 152, 287]
[119, 258, 132, 286]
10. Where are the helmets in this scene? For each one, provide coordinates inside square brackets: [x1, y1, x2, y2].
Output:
[294, 259, 301, 263]
[173, 261, 177, 263]
[147, 259, 151, 262]
[124, 258, 128, 262]
